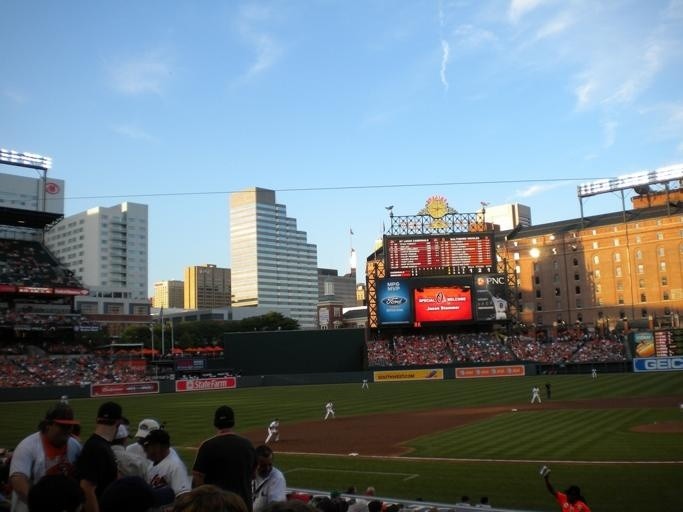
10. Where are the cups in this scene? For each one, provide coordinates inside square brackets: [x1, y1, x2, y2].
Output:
[539, 465, 552, 476]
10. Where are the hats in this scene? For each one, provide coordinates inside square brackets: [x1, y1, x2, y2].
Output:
[134, 418, 160, 438]
[96, 401, 130, 426]
[137, 430, 170, 446]
[98, 475, 175, 511]
[214, 406, 235, 428]
[43, 402, 81, 426]
[115, 423, 132, 441]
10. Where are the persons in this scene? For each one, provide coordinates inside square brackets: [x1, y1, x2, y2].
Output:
[0, 236, 239, 390]
[360, 376, 369, 392]
[530, 384, 542, 405]
[60, 394, 70, 405]
[540, 464, 590, 512]
[366, 329, 629, 367]
[543, 381, 552, 399]
